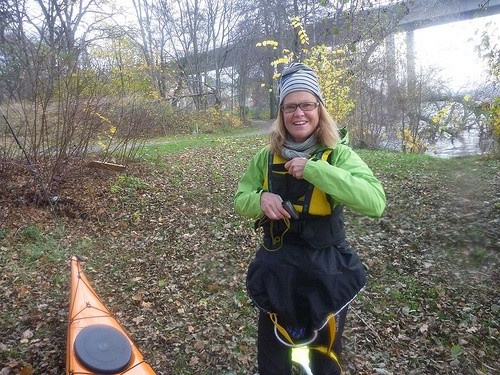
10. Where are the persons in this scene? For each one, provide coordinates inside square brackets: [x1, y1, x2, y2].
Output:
[234, 63, 386, 375]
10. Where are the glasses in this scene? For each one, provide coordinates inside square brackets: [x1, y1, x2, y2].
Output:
[281, 102, 319, 113]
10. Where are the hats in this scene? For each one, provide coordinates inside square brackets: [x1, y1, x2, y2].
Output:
[278, 63, 325, 106]
